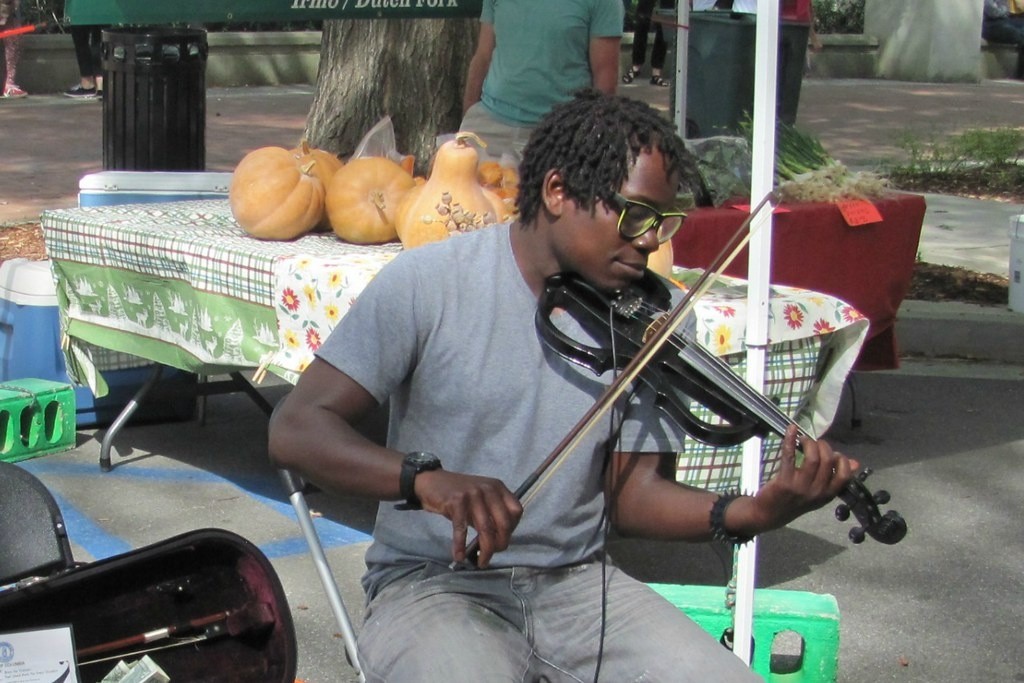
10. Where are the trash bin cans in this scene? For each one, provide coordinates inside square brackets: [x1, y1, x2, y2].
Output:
[659, 10, 814, 145]
[100, 27, 208, 171]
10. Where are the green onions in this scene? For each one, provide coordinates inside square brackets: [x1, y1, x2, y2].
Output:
[737, 111, 891, 202]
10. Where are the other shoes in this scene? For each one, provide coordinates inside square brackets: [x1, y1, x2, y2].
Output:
[95, 90, 103, 99]
[62, 84, 95, 96]
[2, 87, 28, 97]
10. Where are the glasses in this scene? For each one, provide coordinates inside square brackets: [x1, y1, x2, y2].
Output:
[604, 189, 687, 243]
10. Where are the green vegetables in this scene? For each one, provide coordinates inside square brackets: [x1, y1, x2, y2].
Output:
[677, 134, 754, 209]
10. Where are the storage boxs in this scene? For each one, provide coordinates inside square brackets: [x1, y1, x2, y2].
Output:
[75, 169, 234, 208]
[0, 256, 195, 426]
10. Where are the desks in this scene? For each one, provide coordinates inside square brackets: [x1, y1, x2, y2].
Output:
[38, 195, 877, 496]
[663, 182, 939, 424]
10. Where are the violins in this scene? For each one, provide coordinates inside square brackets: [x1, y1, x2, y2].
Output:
[534, 265, 908, 545]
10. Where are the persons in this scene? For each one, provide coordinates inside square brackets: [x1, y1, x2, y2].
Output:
[776, 0, 814, 130]
[619, 0, 675, 85]
[268, 88, 860, 683]
[0, 0, 30, 98]
[62, 24, 111, 101]
[459, 0, 625, 155]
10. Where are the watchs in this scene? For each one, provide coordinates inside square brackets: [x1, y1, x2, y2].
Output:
[394, 452, 441, 512]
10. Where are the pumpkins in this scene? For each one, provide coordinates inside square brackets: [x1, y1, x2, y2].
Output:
[646, 239, 674, 279]
[226, 131, 519, 250]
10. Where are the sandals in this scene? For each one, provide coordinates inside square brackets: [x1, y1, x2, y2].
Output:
[649, 75, 669, 87]
[623, 69, 640, 84]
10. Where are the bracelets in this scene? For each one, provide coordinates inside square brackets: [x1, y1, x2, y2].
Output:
[710, 489, 759, 551]
